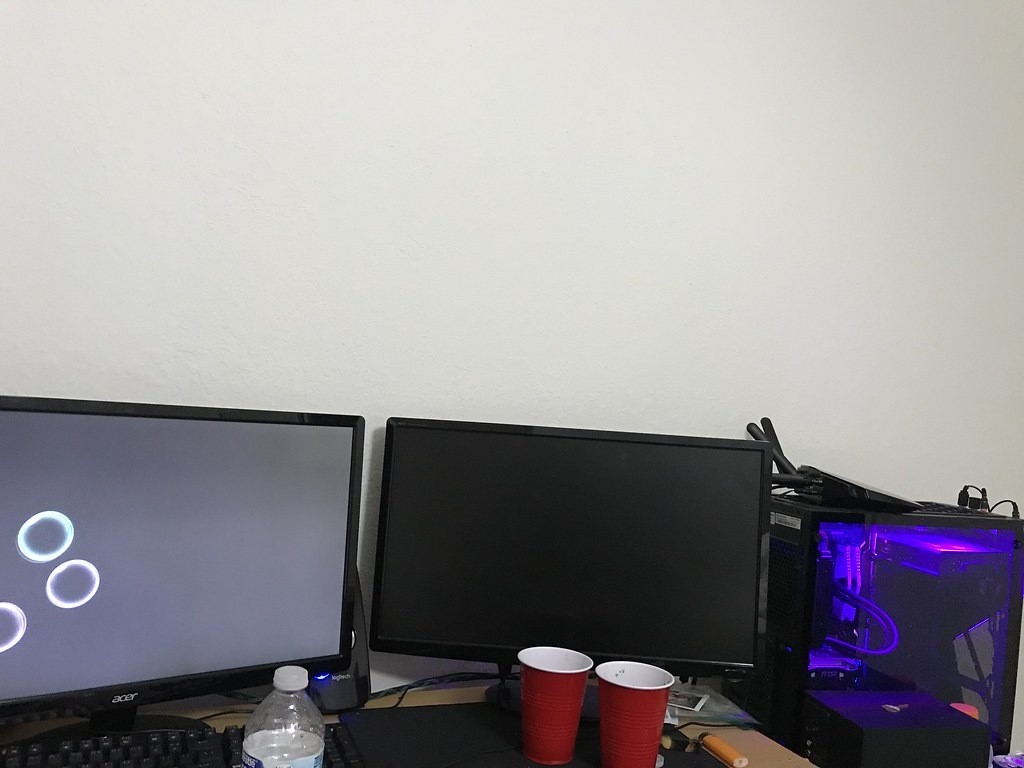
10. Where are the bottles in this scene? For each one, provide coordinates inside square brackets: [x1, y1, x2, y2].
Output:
[242, 666, 325, 768]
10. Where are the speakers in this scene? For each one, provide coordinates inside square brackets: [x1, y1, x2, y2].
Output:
[305, 568, 363, 714]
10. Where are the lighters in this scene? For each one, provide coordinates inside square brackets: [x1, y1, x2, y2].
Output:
[700, 731, 748, 768]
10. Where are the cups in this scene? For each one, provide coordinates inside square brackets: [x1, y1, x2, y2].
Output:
[595, 661, 674, 768]
[517, 646, 593, 765]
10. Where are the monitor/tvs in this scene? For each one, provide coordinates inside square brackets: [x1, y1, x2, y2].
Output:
[0, 395, 364, 747]
[368, 417, 773, 720]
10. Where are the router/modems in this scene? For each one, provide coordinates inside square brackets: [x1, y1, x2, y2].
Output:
[746, 417, 924, 514]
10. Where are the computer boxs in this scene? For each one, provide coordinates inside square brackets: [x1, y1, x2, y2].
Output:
[721, 495, 1024, 757]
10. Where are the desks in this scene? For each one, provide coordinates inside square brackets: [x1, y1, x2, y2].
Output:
[0, 681, 816, 768]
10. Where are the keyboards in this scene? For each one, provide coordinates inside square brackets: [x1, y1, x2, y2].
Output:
[0, 720, 364, 768]
